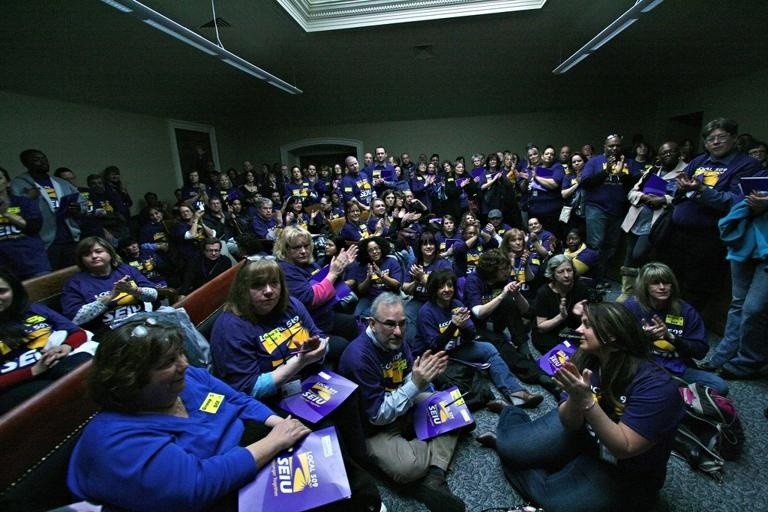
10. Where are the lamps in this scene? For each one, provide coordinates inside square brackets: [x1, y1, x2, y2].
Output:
[101, 0, 305, 100]
[551, 0, 666, 77]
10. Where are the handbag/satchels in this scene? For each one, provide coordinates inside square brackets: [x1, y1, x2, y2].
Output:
[648, 200, 675, 244]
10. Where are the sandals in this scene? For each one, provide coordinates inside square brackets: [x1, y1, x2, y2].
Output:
[482, 502, 546, 512]
[508, 388, 544, 407]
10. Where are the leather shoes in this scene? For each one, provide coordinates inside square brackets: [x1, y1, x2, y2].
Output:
[475, 430, 497, 450]
[485, 399, 510, 415]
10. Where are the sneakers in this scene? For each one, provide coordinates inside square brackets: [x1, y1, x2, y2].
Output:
[412, 473, 467, 512]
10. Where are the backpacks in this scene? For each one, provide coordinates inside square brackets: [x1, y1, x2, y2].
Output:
[676, 381, 745, 483]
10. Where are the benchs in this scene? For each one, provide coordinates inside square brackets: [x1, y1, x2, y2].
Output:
[0, 256, 256, 512]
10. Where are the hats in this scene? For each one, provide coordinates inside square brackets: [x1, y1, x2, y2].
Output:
[487, 208, 503, 219]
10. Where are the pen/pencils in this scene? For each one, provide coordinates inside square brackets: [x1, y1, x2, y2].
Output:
[444, 391, 469, 408]
[290, 348, 316, 355]
[516, 280, 524, 286]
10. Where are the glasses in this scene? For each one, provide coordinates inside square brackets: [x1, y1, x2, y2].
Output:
[607, 133, 619, 140]
[126, 317, 159, 340]
[499, 265, 511, 273]
[240, 254, 278, 268]
[704, 133, 730, 146]
